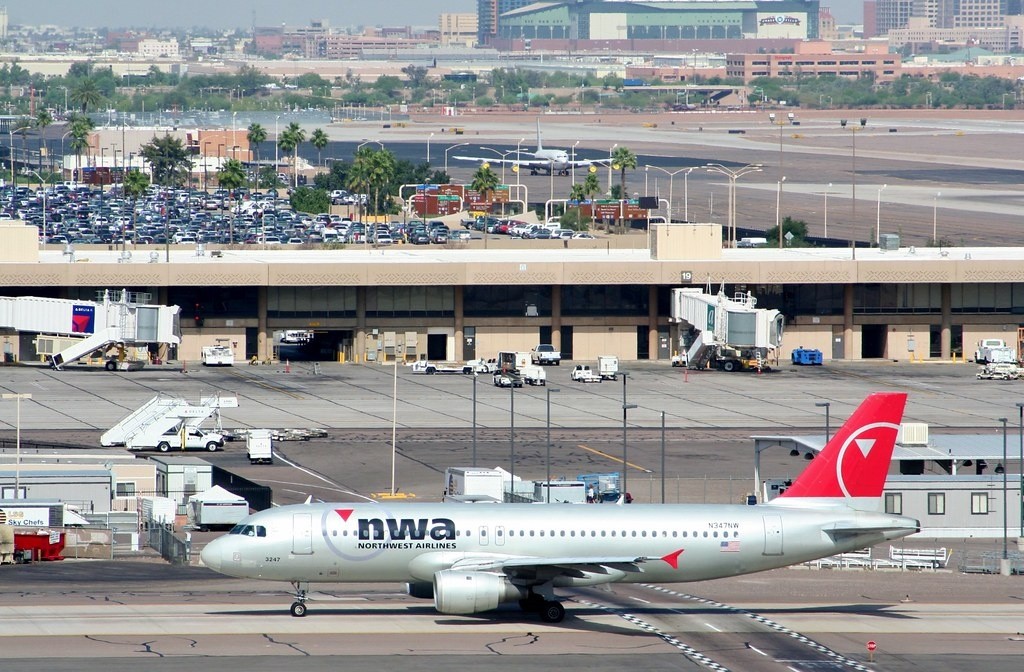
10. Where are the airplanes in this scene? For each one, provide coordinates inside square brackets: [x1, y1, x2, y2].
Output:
[451, 116, 621, 175]
[199, 390, 918, 622]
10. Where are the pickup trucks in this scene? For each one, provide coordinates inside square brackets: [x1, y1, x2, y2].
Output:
[532, 344, 561, 366]
[155, 424, 226, 452]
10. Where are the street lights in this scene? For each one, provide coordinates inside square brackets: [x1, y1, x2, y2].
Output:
[815, 403, 831, 446]
[615, 371, 637, 493]
[26, 169, 62, 246]
[547, 388, 560, 481]
[427, 132, 470, 178]
[769, 113, 795, 248]
[841, 118, 868, 260]
[516, 136, 525, 200]
[998, 403, 1024, 559]
[511, 379, 523, 496]
[100, 141, 240, 181]
[608, 142, 942, 248]
[480, 147, 529, 217]
[571, 140, 579, 194]
[10, 127, 33, 184]
[1, 393, 33, 496]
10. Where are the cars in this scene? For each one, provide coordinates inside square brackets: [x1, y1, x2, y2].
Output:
[0, 182, 597, 245]
